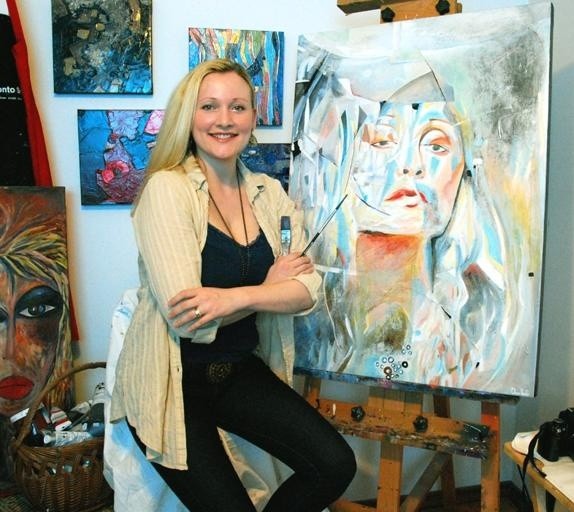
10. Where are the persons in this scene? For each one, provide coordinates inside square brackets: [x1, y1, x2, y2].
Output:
[106, 59, 358, 512]
[290, 47, 519, 387]
[0, 186, 71, 424]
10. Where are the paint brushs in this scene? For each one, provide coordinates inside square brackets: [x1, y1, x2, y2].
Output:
[300, 194, 350, 257]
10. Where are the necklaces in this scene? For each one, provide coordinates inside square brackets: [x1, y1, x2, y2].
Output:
[209, 167, 251, 287]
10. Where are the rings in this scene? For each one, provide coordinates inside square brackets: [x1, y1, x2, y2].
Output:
[195, 309, 201, 317]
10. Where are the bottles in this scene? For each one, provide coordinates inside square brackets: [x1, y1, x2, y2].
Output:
[11, 383, 105, 477]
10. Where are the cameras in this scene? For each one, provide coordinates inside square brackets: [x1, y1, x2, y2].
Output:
[538, 408, 574, 462]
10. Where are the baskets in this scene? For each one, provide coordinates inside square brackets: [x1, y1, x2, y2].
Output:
[2, 362, 115, 512]
